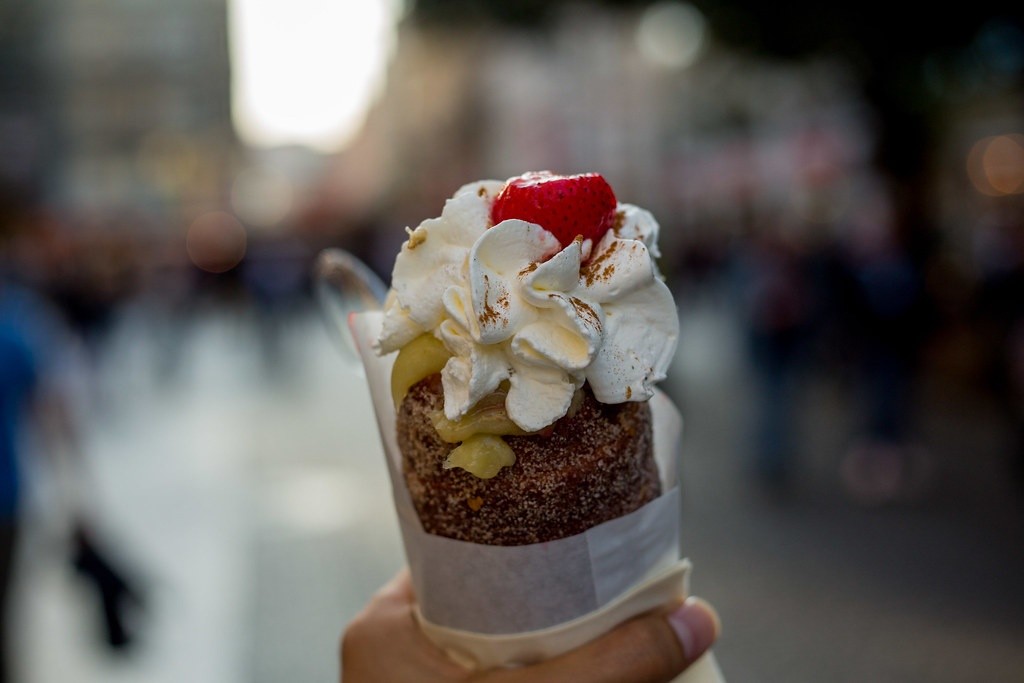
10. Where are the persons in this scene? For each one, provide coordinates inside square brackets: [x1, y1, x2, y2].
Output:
[336, 565, 720, 683]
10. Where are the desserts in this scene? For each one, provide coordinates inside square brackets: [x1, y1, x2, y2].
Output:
[370, 169, 682, 547]
